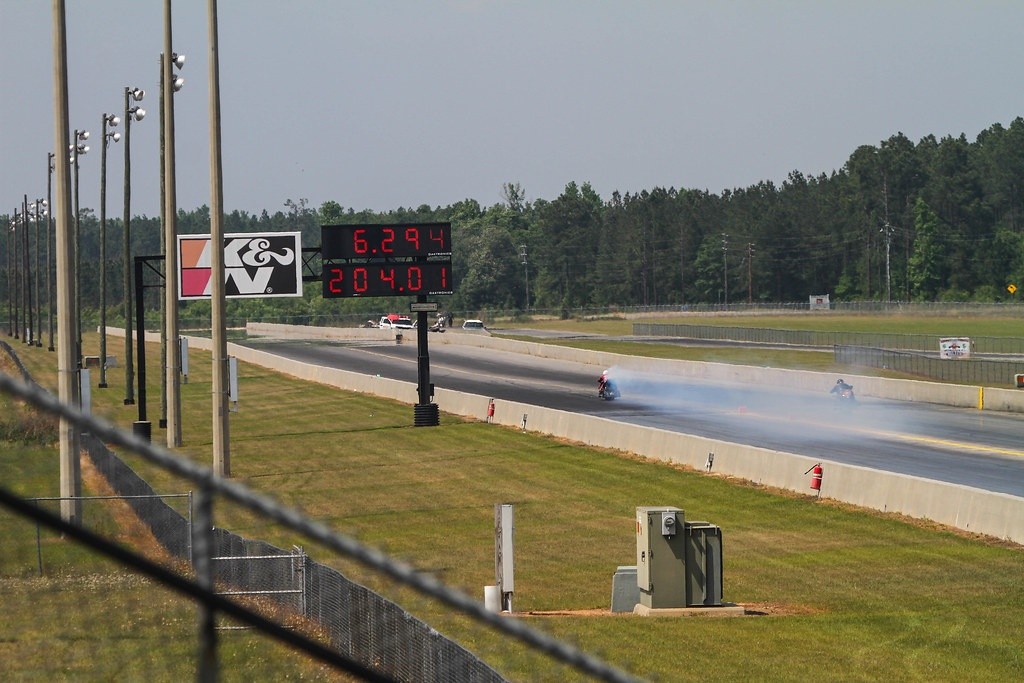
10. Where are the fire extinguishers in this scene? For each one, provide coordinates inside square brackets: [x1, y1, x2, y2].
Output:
[487, 399, 494, 417]
[804, 463, 822, 490]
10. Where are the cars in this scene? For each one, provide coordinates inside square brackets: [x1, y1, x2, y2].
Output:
[462, 319, 486, 330]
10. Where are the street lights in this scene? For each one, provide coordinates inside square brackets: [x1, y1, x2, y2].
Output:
[155, 49, 187, 431]
[6, 129, 91, 370]
[122, 85, 147, 406]
[97, 112, 122, 389]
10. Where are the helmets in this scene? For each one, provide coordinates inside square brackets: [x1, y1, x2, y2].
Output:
[603, 370, 608, 375]
[837, 379, 843, 384]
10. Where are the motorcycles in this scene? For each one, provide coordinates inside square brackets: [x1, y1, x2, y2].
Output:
[596, 376, 620, 402]
[831, 386, 856, 405]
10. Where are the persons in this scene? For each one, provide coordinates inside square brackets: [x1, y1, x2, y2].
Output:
[447, 312, 454, 328]
[596, 369, 617, 397]
[830, 378, 853, 397]
[436, 312, 445, 333]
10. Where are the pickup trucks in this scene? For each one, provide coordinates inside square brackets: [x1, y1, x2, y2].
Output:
[371, 317, 414, 329]
